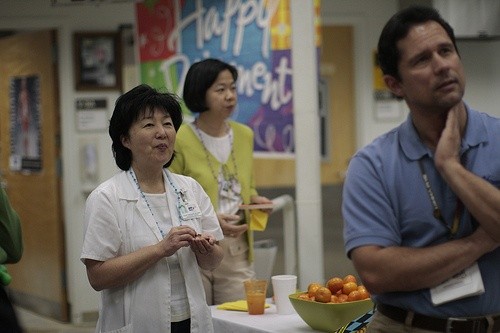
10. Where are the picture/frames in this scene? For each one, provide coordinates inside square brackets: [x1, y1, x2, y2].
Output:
[73, 30, 122, 92]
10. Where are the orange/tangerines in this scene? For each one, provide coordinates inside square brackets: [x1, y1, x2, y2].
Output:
[298, 275, 370, 303]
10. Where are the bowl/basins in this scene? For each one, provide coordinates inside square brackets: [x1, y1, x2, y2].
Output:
[288, 291, 376, 332]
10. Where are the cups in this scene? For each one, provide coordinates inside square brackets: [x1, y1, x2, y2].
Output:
[243, 279, 267, 315]
[271, 275, 297, 315]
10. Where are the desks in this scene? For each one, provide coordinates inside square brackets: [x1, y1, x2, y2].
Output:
[209, 297, 329, 333]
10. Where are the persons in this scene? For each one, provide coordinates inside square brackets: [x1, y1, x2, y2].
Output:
[341, 4, 500, 333]
[0, 184, 25, 333]
[165, 58, 274, 307]
[79, 84, 226, 333]
